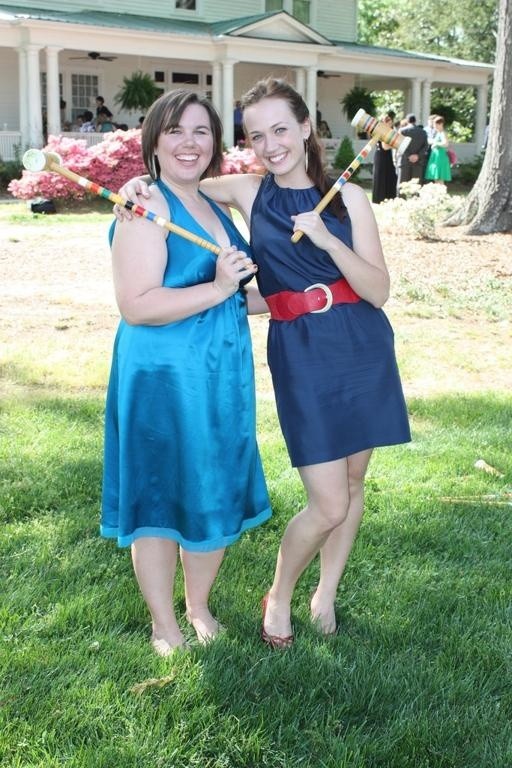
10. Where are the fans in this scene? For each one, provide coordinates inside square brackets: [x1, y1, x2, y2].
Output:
[68, 52, 118, 61]
[317, 71, 341, 79]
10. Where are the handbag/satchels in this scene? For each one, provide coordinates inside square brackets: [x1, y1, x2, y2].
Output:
[447, 150, 456, 165]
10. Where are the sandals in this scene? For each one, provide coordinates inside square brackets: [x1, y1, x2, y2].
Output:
[261, 591, 294, 649]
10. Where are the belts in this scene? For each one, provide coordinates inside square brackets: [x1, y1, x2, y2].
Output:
[264, 277, 361, 321]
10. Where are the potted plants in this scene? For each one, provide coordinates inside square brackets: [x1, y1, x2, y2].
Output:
[338, 86, 382, 139]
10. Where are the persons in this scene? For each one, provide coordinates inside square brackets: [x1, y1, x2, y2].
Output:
[42, 95, 146, 146]
[370, 109, 455, 205]
[234, 100, 245, 146]
[112, 77, 414, 653]
[316, 101, 322, 138]
[99, 89, 270, 658]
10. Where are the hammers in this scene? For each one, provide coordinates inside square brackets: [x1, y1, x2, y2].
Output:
[290, 108, 413, 245]
[21, 148, 260, 274]
